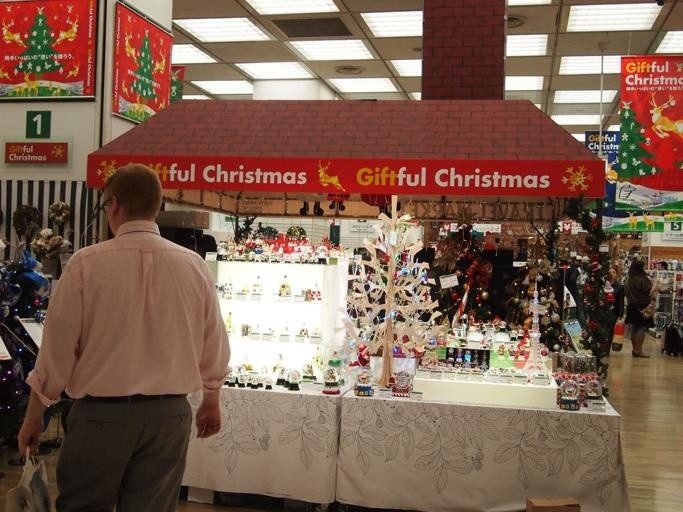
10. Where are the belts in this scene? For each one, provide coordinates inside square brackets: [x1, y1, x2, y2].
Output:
[82, 393, 189, 403]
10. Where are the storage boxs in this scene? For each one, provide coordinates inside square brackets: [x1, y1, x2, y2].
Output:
[365, 350, 420, 381]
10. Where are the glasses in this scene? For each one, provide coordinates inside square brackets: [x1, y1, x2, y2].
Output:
[101, 198, 112, 213]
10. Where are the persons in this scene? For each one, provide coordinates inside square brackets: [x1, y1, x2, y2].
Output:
[560, 256, 669, 358]
[17, 162, 230, 512]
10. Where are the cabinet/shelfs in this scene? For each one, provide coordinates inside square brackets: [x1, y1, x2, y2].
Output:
[643, 268, 682, 340]
[210, 251, 352, 380]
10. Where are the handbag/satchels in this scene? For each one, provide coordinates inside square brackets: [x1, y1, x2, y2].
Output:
[611, 316, 625, 352]
[5, 445, 52, 507]
[639, 300, 656, 320]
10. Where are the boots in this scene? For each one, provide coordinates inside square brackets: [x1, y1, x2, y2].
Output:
[633, 335, 650, 358]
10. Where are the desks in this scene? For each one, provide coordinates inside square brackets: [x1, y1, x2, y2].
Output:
[180, 380, 353, 511]
[332, 381, 630, 509]
[421, 318, 558, 385]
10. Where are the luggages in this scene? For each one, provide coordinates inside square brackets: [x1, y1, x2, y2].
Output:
[667, 326, 683, 353]
[660, 324, 669, 353]
